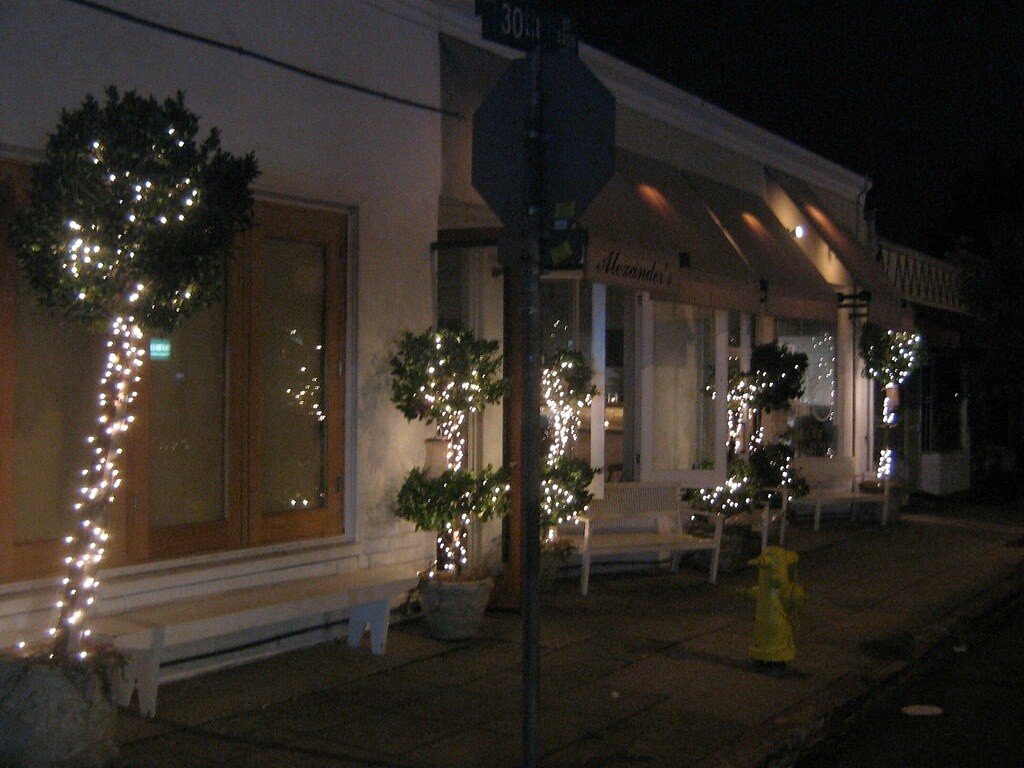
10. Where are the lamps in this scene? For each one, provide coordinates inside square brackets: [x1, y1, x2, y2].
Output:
[791, 225, 803, 239]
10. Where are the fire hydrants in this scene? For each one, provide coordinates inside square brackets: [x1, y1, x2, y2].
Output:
[747, 545, 808, 673]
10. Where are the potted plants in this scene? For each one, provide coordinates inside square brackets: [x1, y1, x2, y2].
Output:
[0, 83, 265, 768]
[387, 322, 519, 642]
[859, 322, 927, 412]
[487, 338, 602, 611]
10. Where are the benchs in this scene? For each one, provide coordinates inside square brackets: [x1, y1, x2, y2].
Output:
[669, 481, 787, 584]
[78, 564, 418, 720]
[544, 479, 723, 595]
[789, 457, 888, 532]
